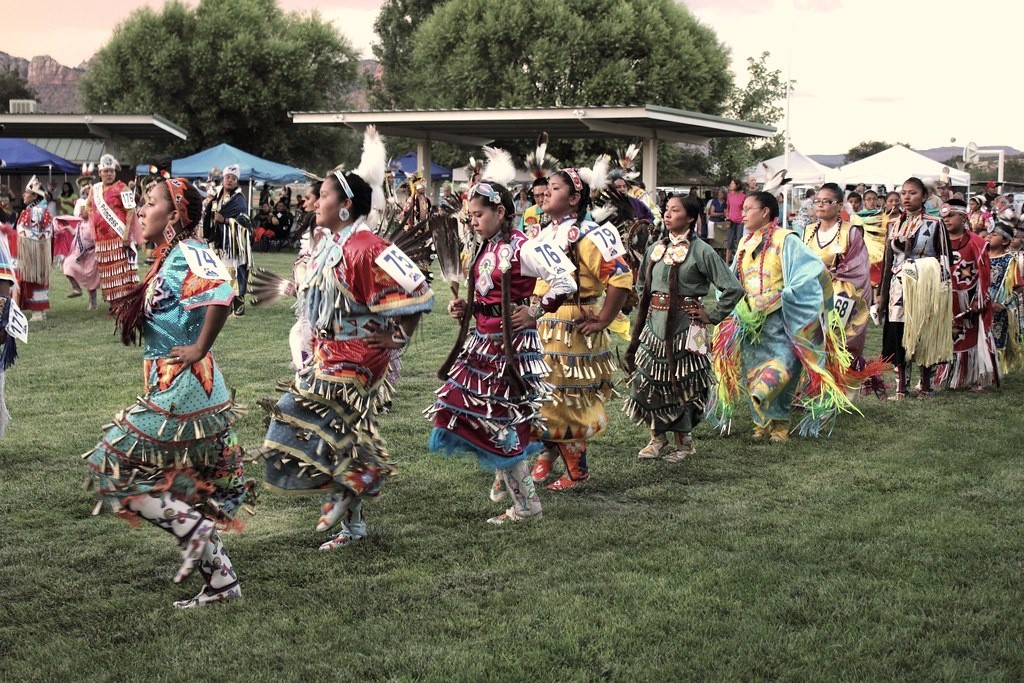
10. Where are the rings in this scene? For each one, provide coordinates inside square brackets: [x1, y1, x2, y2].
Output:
[448, 303, 452, 313]
[176, 356, 182, 364]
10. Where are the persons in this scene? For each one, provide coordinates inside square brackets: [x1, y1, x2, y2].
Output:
[706, 191, 866, 441]
[876, 177, 953, 401]
[624, 192, 744, 464]
[421, 180, 576, 525]
[800, 183, 872, 420]
[0, 230, 28, 438]
[530, 169, 633, 489]
[0, 153, 1024, 391]
[81, 178, 259, 609]
[261, 171, 431, 552]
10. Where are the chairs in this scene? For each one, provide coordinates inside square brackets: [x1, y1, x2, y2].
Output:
[255, 205, 303, 253]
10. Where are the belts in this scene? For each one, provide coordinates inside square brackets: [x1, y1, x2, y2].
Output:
[650, 293, 701, 309]
[562, 296, 598, 304]
[474, 296, 529, 318]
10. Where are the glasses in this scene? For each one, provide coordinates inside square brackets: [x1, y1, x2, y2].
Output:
[742, 206, 759, 212]
[814, 198, 840, 205]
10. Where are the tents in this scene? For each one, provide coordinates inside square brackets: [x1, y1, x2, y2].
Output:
[740, 144, 971, 213]
[0, 139, 82, 194]
[386, 151, 451, 183]
[136, 143, 306, 185]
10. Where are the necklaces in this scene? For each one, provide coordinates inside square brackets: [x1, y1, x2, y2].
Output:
[735, 215, 778, 297]
[956, 230, 965, 247]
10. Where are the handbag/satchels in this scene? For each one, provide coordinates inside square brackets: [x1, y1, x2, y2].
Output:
[685, 315, 707, 354]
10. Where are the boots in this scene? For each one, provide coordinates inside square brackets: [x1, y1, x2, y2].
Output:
[314, 488, 362, 533]
[663, 428, 696, 463]
[175, 531, 245, 606]
[88, 289, 97, 310]
[918, 363, 945, 400]
[66, 276, 82, 297]
[120, 489, 219, 584]
[320, 500, 366, 552]
[887, 361, 911, 400]
[547, 439, 590, 489]
[639, 425, 668, 458]
[532, 446, 559, 481]
[487, 459, 544, 524]
[491, 467, 521, 502]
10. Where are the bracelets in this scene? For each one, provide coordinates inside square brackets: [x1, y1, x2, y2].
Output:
[392, 323, 410, 345]
